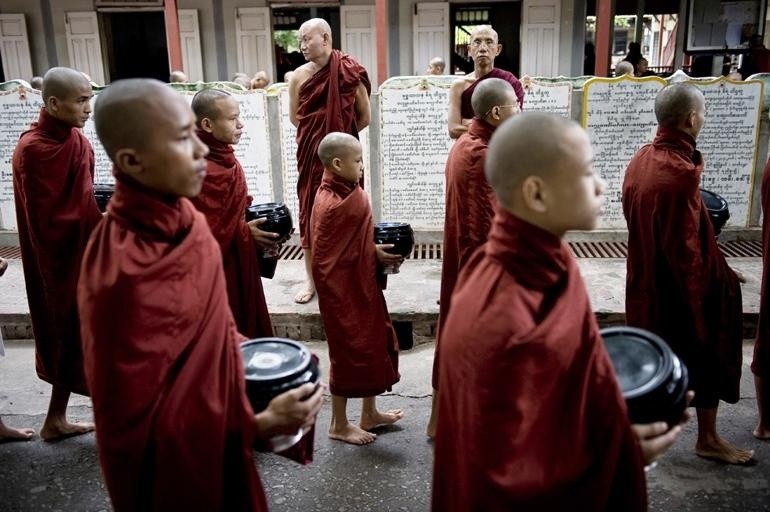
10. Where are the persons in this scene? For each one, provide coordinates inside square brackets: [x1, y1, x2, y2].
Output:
[448, 26, 524, 137]
[310, 132, 403, 445]
[288, 17, 371, 303]
[11, 66, 103, 442]
[188, 87, 294, 340]
[751, 156, 770, 440]
[431, 111, 694, 512]
[584, 33, 770, 78]
[428, 77, 521, 438]
[623, 83, 755, 466]
[0, 258, 35, 442]
[425, 58, 445, 76]
[163, 70, 269, 87]
[77, 78, 323, 512]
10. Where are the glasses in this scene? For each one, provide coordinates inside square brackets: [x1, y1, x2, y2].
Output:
[482, 102, 521, 119]
